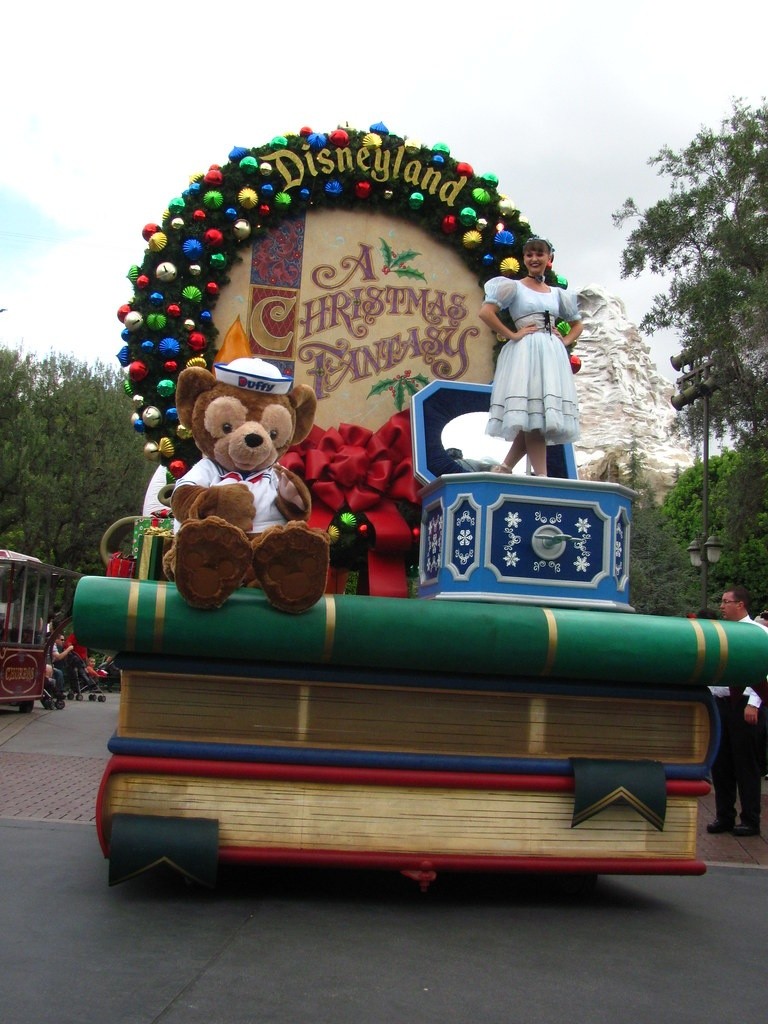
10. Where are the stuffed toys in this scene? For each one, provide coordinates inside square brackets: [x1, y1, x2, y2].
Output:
[162, 357, 330, 614]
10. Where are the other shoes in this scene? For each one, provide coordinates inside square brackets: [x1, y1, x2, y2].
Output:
[492, 463, 513, 474]
[707, 818, 735, 832]
[734, 823, 760, 836]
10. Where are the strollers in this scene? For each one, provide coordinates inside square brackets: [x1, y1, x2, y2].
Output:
[42, 677, 65, 710]
[62, 642, 107, 702]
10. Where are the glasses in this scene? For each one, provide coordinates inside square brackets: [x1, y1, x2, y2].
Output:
[720, 599, 739, 603]
[60, 639, 65, 641]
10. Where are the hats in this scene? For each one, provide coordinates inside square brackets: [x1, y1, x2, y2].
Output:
[526, 237, 553, 250]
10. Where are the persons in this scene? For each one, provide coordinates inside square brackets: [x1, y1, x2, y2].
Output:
[698, 606, 768, 626]
[478, 238, 584, 479]
[706, 585, 768, 836]
[36, 616, 124, 700]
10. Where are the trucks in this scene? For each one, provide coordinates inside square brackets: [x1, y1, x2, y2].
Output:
[0, 549, 87, 713]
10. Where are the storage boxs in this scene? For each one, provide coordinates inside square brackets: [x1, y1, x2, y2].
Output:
[106, 518, 173, 582]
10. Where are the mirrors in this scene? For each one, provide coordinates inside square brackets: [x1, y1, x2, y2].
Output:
[441, 412, 534, 476]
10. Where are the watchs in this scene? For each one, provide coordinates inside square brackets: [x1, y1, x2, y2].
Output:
[749, 704, 757, 709]
